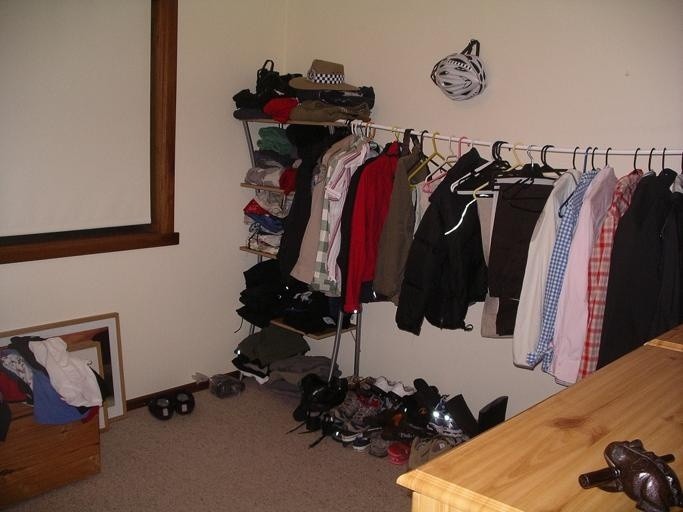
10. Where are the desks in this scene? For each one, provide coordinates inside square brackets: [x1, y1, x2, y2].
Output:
[644, 321, 683, 355]
[393, 340, 682, 510]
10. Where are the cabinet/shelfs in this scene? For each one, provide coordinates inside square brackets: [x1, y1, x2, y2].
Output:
[236, 113, 367, 392]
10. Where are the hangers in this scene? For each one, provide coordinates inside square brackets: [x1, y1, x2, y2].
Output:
[451, 141, 683, 221]
[329, 119, 474, 194]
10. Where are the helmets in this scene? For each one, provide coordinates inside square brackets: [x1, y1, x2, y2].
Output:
[428, 36, 488, 102]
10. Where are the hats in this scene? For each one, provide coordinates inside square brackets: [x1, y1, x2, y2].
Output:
[288, 57, 364, 94]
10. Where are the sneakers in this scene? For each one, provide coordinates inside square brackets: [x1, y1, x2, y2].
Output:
[175, 389, 200, 415]
[289, 365, 511, 474]
[211, 377, 245, 399]
[147, 395, 174, 421]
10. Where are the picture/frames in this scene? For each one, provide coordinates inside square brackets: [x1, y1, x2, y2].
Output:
[0, 311, 132, 426]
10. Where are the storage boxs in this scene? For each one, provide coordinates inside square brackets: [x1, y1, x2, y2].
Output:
[0, 401, 103, 510]
